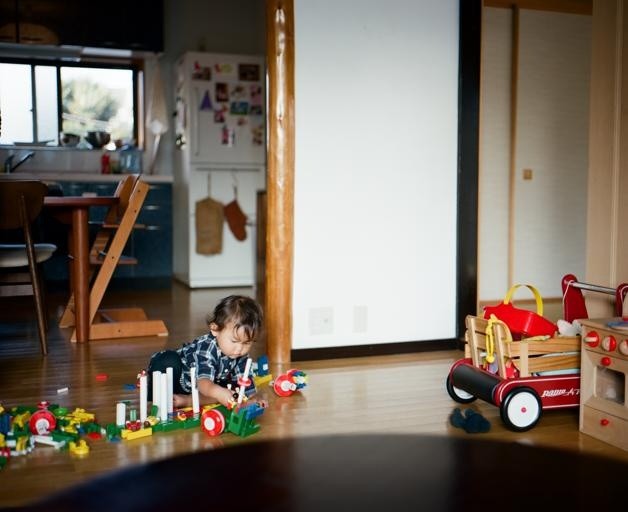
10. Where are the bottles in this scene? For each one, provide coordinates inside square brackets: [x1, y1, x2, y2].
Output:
[101, 150, 111, 174]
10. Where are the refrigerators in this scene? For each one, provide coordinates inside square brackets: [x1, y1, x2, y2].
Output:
[171, 51, 266, 288]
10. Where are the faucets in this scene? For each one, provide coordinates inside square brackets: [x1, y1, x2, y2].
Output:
[10, 151, 36, 173]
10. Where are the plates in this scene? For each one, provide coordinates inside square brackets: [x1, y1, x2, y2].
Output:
[14, 142, 48, 146]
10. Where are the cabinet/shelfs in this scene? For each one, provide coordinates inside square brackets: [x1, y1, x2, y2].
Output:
[579, 317, 628, 449]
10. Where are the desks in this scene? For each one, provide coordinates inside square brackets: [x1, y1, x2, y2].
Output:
[44, 195, 120, 344]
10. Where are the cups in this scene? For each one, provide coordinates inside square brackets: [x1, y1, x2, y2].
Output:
[112, 161, 122, 173]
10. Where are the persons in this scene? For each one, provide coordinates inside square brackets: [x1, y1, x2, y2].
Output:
[146, 295, 264, 407]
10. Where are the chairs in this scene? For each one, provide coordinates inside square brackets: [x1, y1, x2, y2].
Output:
[0, 180, 58, 356]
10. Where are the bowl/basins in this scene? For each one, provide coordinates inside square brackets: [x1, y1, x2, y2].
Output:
[59, 131, 110, 150]
